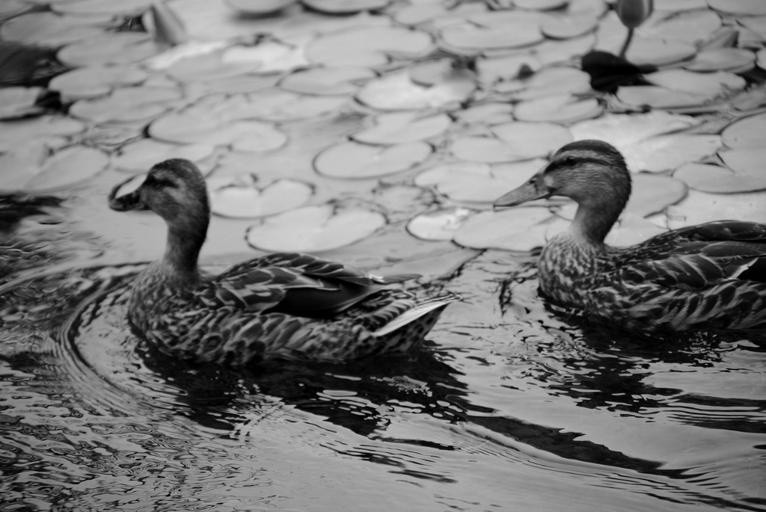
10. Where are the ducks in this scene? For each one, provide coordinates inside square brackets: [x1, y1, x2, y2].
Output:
[107, 156, 470, 366]
[478, 138, 765, 339]
